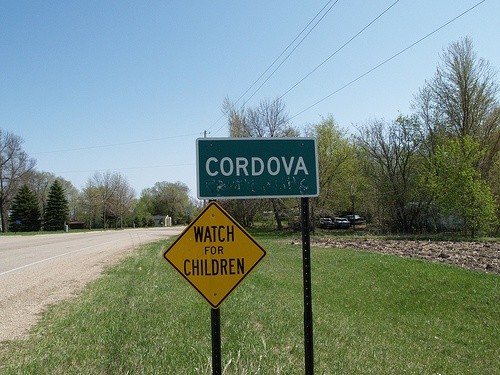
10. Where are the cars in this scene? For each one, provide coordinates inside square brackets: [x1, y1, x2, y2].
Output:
[333, 216, 350, 229]
[317, 217, 336, 230]
[342, 215, 365, 225]
